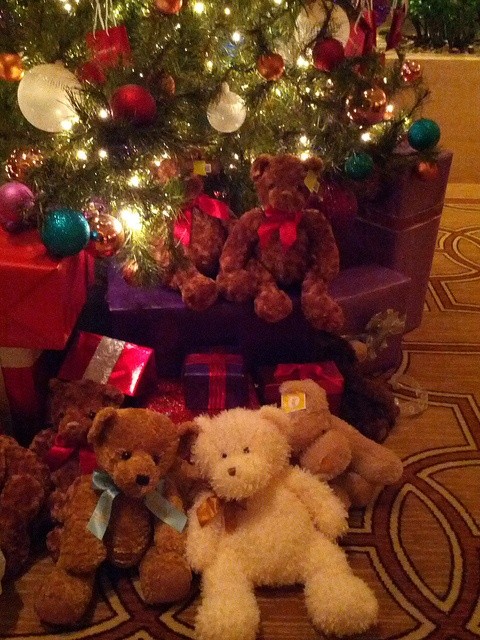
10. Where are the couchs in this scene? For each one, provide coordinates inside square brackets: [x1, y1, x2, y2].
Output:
[106, 134, 453, 377]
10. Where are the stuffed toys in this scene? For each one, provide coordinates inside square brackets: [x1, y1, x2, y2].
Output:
[23, 378, 125, 486]
[138, 150, 239, 311]
[260, 379, 405, 511]
[0, 434, 50, 587]
[219, 150, 346, 334]
[29, 405, 198, 627]
[183, 403, 379, 639]
